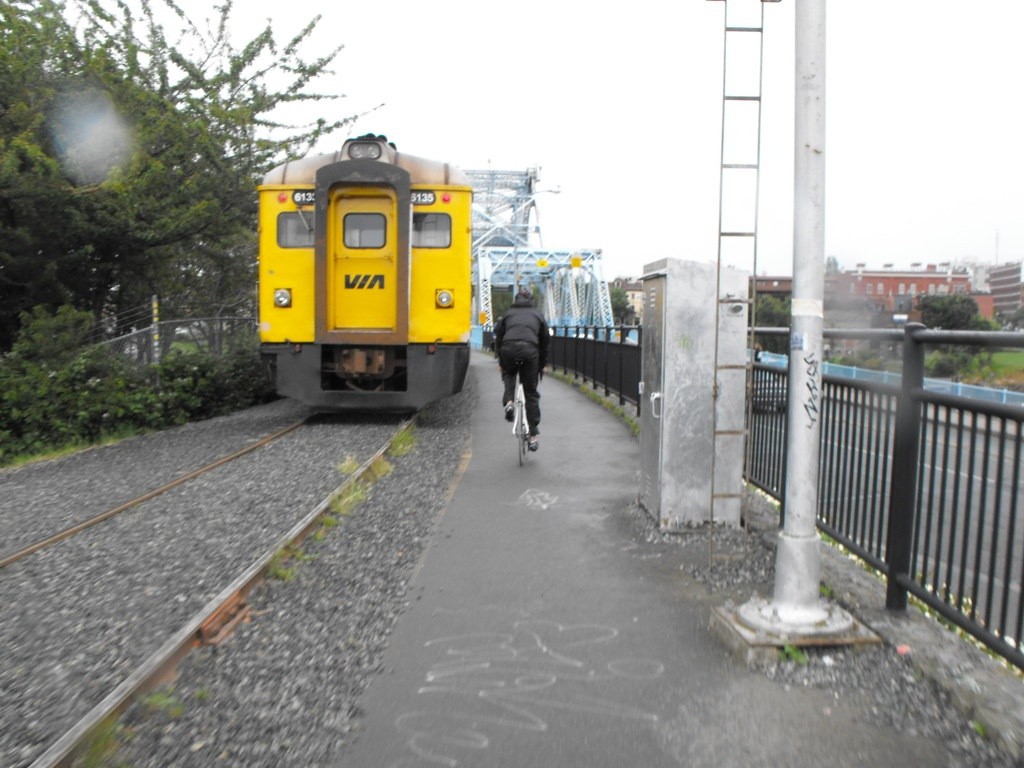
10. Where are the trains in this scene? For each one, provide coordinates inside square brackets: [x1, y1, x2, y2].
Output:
[254, 133, 473, 413]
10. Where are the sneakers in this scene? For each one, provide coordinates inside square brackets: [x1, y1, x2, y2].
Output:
[505, 401, 514, 422]
[528, 440, 540, 451]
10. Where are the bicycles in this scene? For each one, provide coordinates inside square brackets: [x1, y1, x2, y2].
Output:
[506, 359, 544, 466]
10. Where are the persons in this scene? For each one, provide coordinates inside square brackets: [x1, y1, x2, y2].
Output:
[494, 286, 550, 452]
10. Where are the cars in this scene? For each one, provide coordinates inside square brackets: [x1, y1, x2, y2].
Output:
[753, 369, 788, 412]
